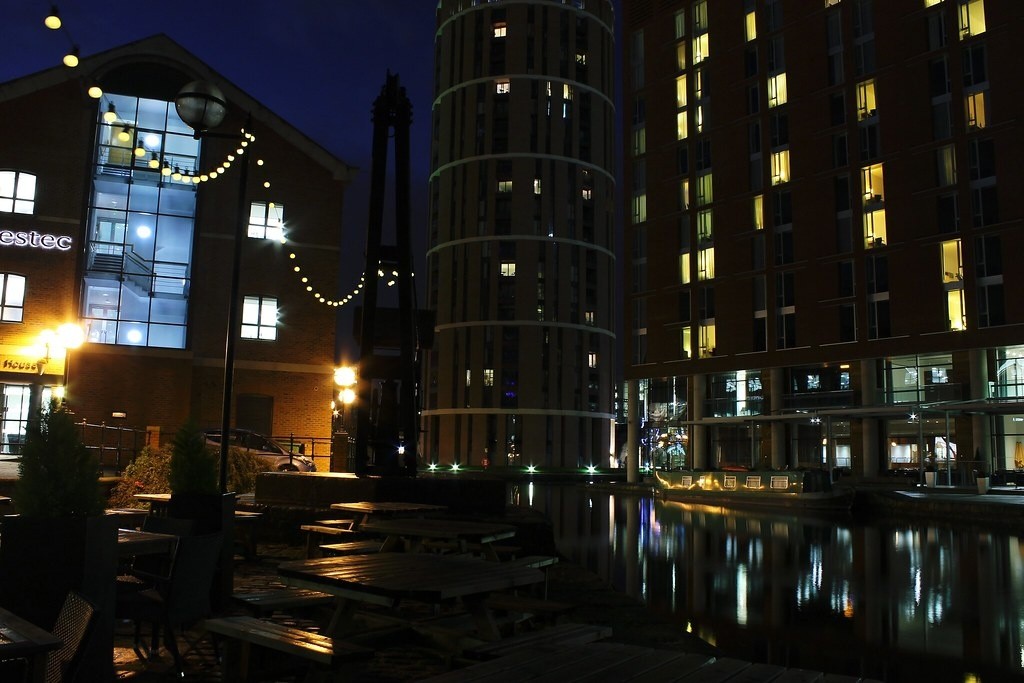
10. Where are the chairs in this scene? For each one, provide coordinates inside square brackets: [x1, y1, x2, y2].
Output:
[116, 515, 197, 664]
[48, 592, 104, 683]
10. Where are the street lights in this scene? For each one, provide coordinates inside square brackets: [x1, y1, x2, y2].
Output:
[176, 80, 255, 491]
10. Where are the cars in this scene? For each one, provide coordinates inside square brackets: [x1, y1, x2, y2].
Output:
[200, 430, 318, 471]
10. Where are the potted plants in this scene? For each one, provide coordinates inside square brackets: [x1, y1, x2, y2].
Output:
[136, 417, 225, 621]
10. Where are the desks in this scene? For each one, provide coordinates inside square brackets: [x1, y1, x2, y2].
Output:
[330, 501, 450, 552]
[415, 640, 878, 683]
[271, 550, 547, 683]
[358, 518, 517, 562]
[116, 527, 188, 676]
[0, 606, 64, 683]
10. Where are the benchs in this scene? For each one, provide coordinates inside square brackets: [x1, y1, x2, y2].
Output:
[318, 538, 411, 556]
[316, 518, 353, 557]
[301, 525, 360, 560]
[510, 555, 559, 569]
[477, 594, 565, 626]
[426, 542, 522, 561]
[231, 589, 334, 683]
[463, 621, 612, 662]
[203, 616, 375, 683]
